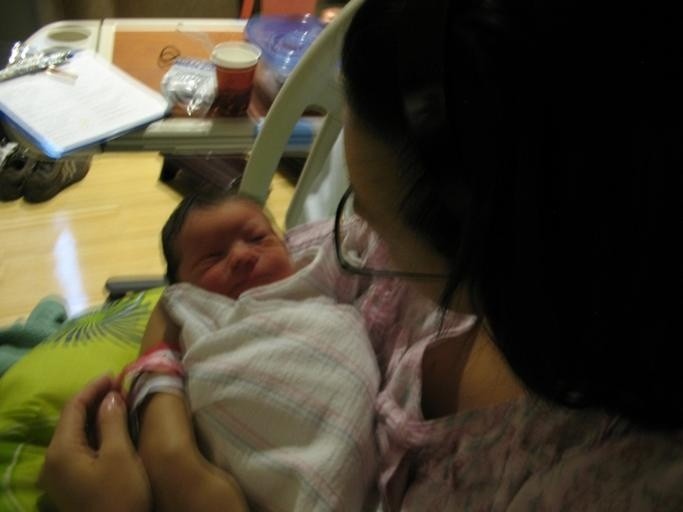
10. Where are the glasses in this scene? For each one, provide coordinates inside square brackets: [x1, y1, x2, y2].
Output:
[332, 182, 461, 298]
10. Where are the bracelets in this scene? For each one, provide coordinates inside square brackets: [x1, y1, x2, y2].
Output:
[116, 345, 186, 413]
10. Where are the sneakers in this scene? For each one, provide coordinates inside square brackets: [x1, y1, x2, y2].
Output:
[0, 143, 39, 203]
[22, 154, 90, 205]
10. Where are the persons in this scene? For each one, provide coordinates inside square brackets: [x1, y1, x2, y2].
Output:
[161, 191, 381, 511]
[41, 0, 683, 512]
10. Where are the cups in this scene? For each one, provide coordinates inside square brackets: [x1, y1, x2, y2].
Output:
[211, 42, 262, 114]
